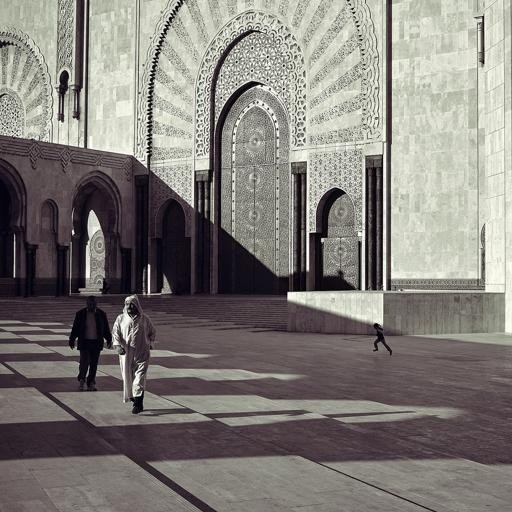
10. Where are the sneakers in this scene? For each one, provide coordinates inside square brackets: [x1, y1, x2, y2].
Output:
[87, 383, 98, 391]
[79, 381, 84, 389]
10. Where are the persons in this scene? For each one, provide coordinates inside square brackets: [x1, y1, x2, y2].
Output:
[111, 292, 157, 416]
[68, 294, 112, 393]
[372, 323, 393, 357]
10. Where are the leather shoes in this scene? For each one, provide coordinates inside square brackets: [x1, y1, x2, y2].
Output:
[131, 404, 143, 414]
